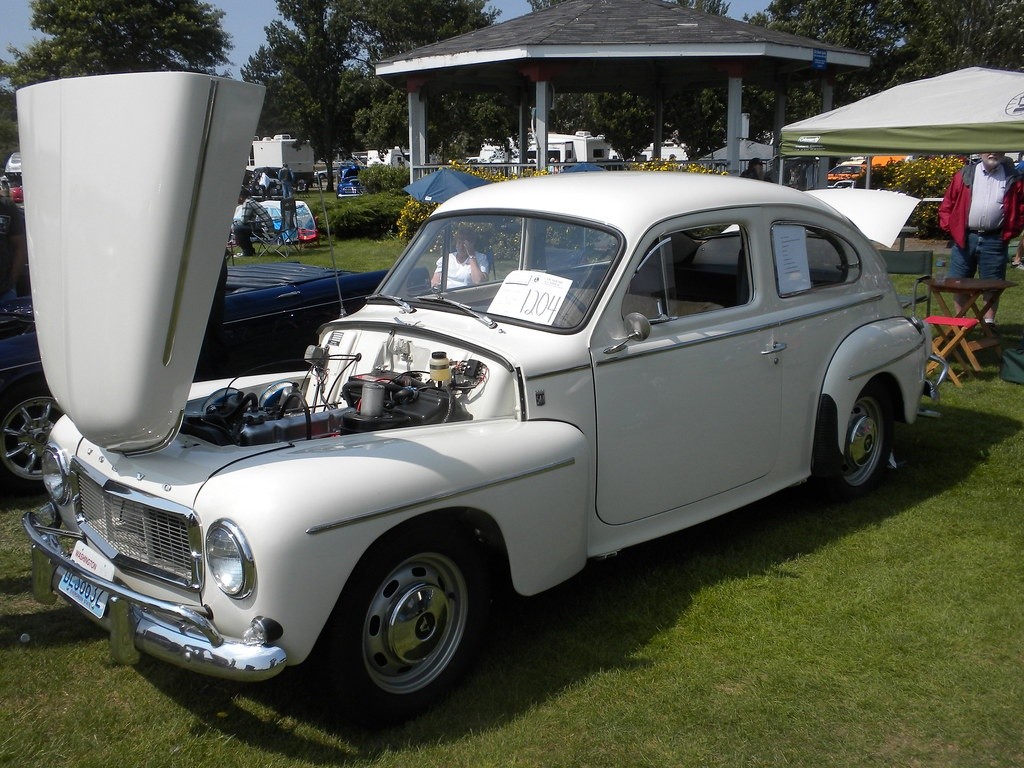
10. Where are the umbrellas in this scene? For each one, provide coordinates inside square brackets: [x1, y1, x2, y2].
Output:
[401, 168, 492, 205]
[560, 162, 602, 173]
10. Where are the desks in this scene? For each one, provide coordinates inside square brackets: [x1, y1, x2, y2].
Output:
[923, 277, 1014, 371]
[898, 227, 919, 251]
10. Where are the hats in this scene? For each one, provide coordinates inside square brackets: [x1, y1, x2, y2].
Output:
[750, 158, 767, 164]
[669, 154, 677, 158]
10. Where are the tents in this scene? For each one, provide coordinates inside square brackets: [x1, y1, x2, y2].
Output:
[777, 66, 1024, 191]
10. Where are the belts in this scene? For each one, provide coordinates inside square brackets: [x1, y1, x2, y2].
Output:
[968, 229, 1002, 237]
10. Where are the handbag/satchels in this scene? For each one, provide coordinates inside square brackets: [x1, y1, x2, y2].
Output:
[1000, 346, 1024, 385]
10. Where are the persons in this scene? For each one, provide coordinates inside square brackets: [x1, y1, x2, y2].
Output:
[430, 228, 490, 291]
[739, 158, 766, 181]
[258, 168, 276, 201]
[278, 163, 294, 200]
[937, 151, 1023, 337]
[233, 187, 275, 257]
[337, 166, 343, 184]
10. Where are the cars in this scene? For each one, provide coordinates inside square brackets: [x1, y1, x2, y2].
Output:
[1, 257, 434, 498]
[821, 161, 866, 192]
[18, 71, 954, 732]
[240, 134, 410, 199]
[0, 150, 24, 243]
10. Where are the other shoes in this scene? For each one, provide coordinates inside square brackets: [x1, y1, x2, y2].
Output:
[984, 322, 1001, 337]
[233, 252, 255, 258]
[1011, 262, 1024, 270]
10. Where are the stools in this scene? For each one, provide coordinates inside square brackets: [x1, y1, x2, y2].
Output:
[923, 316, 981, 387]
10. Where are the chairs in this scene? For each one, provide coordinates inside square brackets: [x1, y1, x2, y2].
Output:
[296, 179, 313, 198]
[298, 216, 321, 247]
[551, 236, 743, 327]
[247, 197, 301, 259]
[879, 249, 933, 318]
[477, 247, 497, 280]
